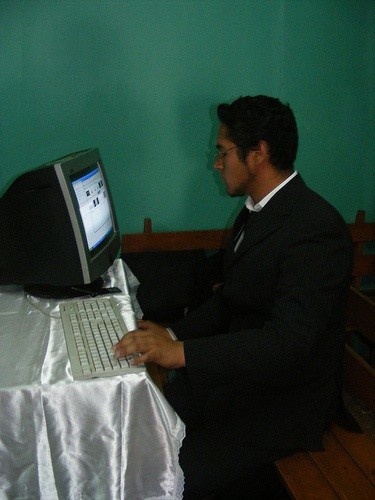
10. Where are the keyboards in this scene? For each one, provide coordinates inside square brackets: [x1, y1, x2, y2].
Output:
[59, 298, 147, 380]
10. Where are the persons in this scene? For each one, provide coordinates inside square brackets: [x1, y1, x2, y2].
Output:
[112, 94, 356, 500]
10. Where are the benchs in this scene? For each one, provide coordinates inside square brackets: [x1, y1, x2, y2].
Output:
[271, 284, 375, 500]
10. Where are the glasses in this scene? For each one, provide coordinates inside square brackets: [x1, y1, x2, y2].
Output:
[215, 145, 236, 160]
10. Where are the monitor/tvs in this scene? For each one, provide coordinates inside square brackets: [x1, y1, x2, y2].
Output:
[0, 147, 121, 287]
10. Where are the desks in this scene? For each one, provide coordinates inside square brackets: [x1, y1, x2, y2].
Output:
[0, 257, 147, 500]
